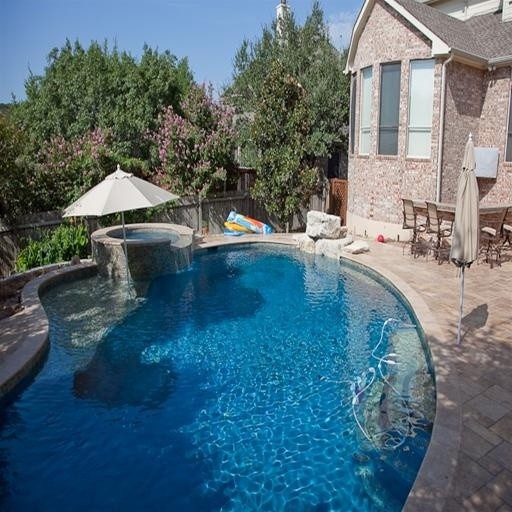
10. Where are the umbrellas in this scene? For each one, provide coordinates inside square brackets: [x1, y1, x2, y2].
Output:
[62, 164, 180, 286]
[449, 132, 480, 342]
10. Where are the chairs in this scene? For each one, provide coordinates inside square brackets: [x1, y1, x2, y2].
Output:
[401, 197, 511, 270]
